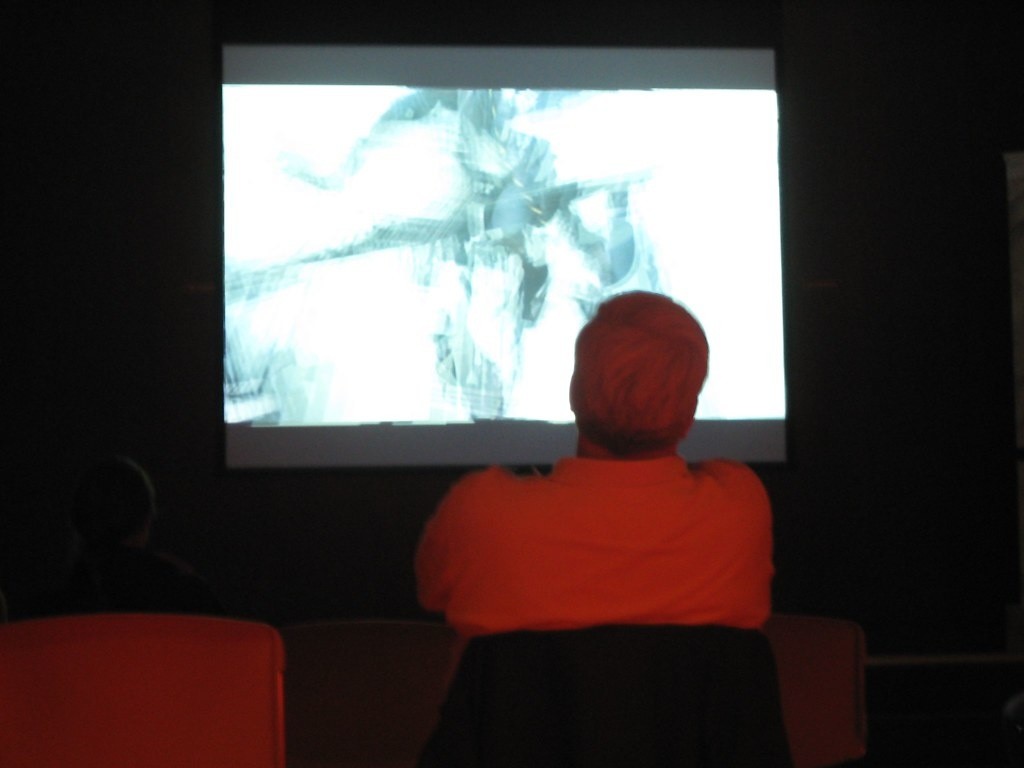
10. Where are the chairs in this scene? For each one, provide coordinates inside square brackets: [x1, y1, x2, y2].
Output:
[0, 615, 867, 768]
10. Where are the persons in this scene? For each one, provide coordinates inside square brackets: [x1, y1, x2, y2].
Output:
[417, 294, 774, 680]
[34, 458, 222, 614]
